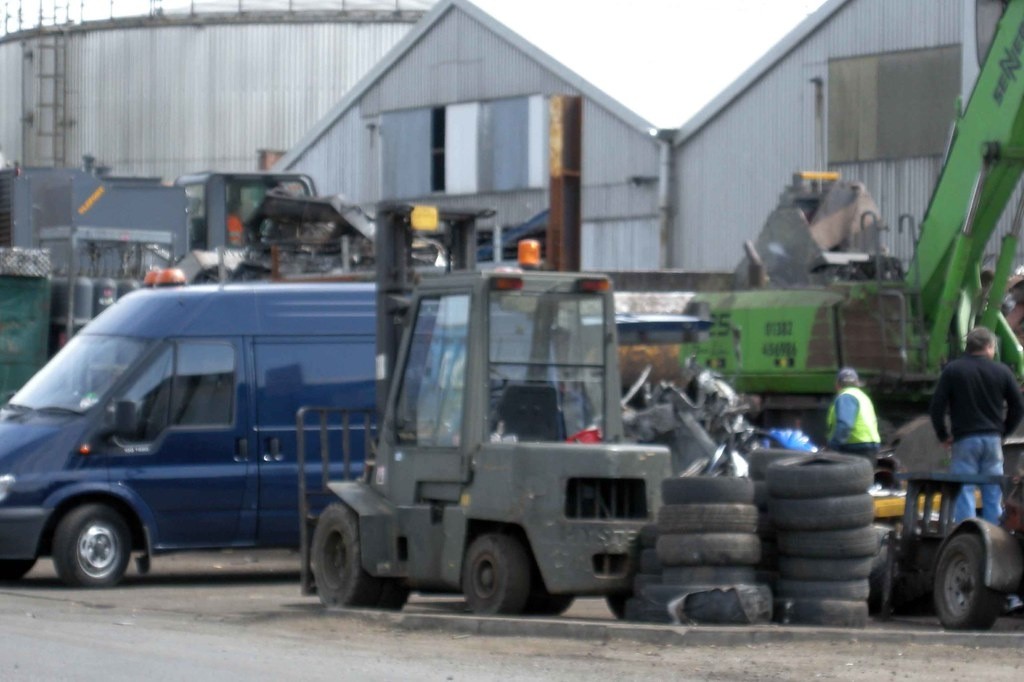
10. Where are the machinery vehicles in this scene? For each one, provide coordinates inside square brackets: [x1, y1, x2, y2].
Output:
[293, 197, 672, 617]
[679, 1, 1024, 461]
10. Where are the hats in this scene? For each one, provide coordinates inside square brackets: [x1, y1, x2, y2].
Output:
[838, 369, 858, 383]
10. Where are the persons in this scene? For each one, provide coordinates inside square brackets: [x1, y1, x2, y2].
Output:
[824, 367, 881, 469]
[929, 327, 1024, 525]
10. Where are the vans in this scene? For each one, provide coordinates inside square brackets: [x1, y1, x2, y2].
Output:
[0, 284, 585, 590]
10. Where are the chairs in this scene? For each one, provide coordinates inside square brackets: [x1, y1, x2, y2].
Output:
[488, 383, 565, 445]
